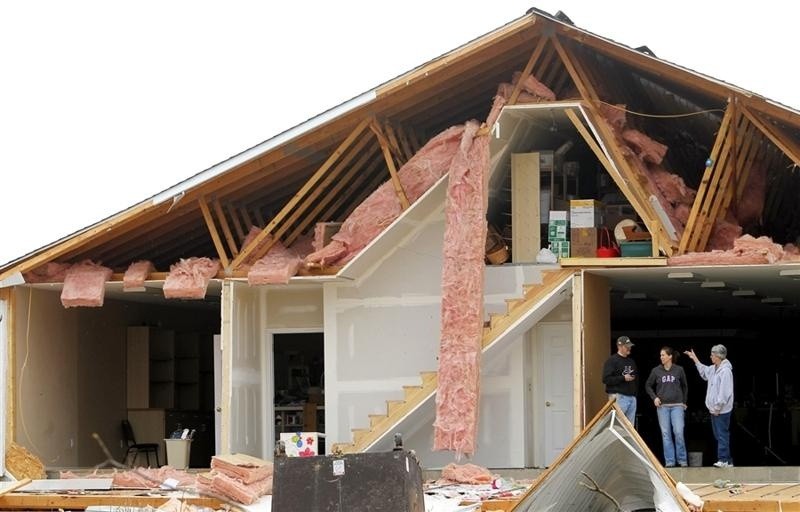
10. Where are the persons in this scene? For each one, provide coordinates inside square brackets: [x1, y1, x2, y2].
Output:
[603, 335, 641, 428]
[683, 344, 734, 468]
[645, 346, 689, 467]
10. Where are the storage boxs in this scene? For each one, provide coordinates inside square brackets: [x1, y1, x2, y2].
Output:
[547, 195, 606, 258]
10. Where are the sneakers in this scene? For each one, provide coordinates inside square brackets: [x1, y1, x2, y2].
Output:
[713, 461, 734, 468]
[664, 462, 688, 467]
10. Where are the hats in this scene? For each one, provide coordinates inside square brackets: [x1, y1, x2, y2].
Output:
[711, 344, 728, 359]
[616, 335, 635, 348]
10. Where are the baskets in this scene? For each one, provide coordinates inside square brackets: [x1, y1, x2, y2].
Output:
[486, 232, 510, 264]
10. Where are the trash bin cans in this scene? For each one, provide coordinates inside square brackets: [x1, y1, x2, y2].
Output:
[163, 439, 193, 470]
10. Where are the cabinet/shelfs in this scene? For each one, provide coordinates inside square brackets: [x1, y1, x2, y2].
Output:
[508, 149, 580, 262]
[128, 326, 201, 469]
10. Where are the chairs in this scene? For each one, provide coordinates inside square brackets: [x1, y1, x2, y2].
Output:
[120, 419, 159, 469]
